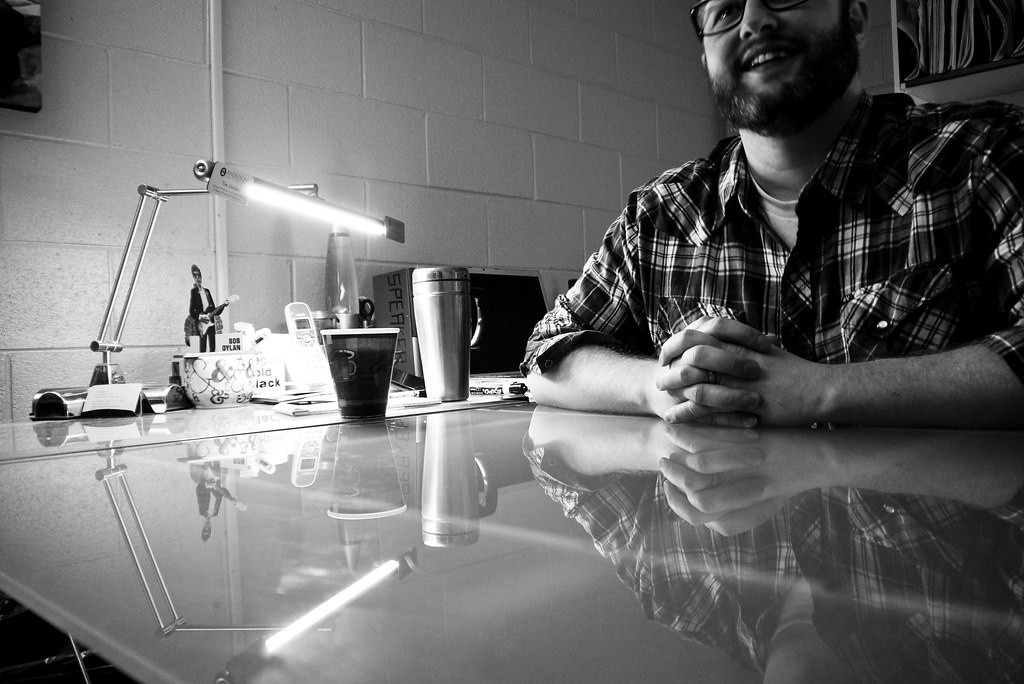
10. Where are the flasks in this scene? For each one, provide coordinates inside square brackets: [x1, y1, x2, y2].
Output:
[420, 412, 487, 548]
[412, 264, 472, 404]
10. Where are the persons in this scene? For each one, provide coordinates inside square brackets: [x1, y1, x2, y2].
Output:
[520, 0, 1024, 429]
[197, 459, 235, 543]
[522, 405, 1023, 683]
[189, 265, 225, 352]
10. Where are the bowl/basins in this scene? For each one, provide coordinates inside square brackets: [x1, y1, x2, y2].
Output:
[179, 350, 260, 410]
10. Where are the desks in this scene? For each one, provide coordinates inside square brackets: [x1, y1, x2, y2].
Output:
[0, 397, 1024, 683]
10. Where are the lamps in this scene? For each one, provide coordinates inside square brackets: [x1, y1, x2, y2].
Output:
[29, 158, 405, 422]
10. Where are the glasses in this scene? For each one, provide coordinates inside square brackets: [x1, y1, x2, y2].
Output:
[690, 0, 806, 42]
[193, 273, 201, 277]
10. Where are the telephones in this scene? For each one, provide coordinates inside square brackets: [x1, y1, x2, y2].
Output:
[284, 301, 331, 387]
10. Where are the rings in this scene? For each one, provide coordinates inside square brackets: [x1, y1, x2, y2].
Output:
[708, 370, 714, 383]
[712, 474, 718, 487]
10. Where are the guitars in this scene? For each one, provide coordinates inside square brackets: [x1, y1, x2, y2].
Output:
[196, 294, 241, 340]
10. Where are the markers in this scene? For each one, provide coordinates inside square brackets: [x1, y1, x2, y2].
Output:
[470, 382, 528, 395]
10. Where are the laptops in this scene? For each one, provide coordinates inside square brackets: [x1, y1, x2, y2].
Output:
[464, 267, 551, 394]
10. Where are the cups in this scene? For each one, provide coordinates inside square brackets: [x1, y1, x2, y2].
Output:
[318, 326, 400, 421]
[326, 424, 408, 521]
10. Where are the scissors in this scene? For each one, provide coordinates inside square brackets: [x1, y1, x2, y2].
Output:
[358, 298, 375, 328]
